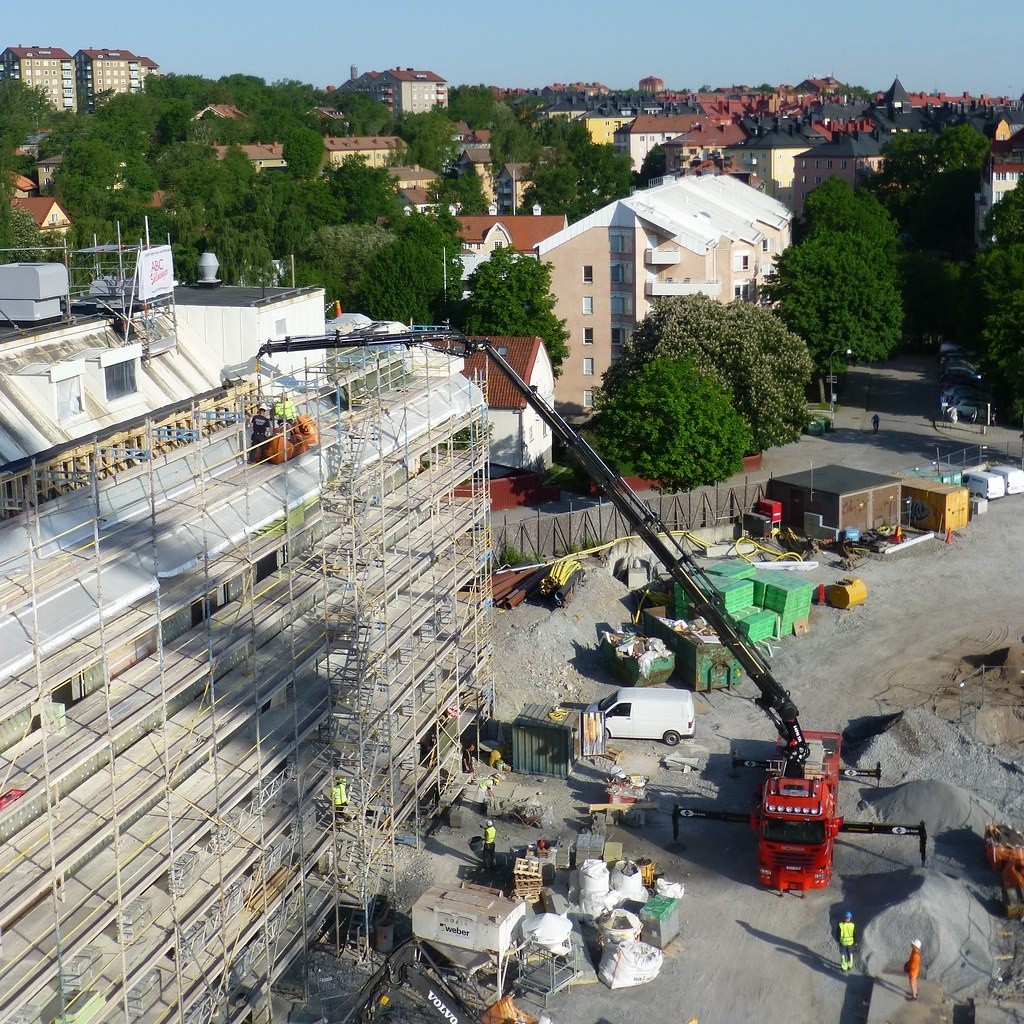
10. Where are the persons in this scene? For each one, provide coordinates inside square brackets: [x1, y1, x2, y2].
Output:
[274, 392, 297, 426]
[478, 779, 499, 796]
[904, 939, 922, 1001]
[330, 776, 348, 830]
[478, 820, 496, 873]
[245, 405, 271, 465]
[836, 911, 857, 973]
[462, 744, 476, 784]
[870, 413, 880, 433]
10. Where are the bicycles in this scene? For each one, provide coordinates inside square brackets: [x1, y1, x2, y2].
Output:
[872, 421, 879, 430]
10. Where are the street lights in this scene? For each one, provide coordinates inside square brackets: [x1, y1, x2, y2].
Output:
[831, 349, 852, 432]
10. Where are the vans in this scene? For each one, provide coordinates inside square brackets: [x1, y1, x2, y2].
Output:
[961, 465, 1024, 502]
[586, 687, 695, 746]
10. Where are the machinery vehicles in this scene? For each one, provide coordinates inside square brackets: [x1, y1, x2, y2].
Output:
[255, 329, 927, 892]
[325, 932, 541, 1024]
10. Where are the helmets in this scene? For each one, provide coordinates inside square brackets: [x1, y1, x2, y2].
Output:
[911, 939, 922, 949]
[334, 776, 342, 781]
[842, 911, 852, 918]
[485, 819, 493, 826]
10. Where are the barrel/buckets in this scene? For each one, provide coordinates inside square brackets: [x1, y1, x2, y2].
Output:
[375, 918, 393, 952]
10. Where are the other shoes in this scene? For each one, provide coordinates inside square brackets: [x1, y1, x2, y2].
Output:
[907, 993, 918, 1001]
[843, 966, 850, 972]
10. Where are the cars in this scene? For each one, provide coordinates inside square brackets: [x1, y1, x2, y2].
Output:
[951, 399, 985, 418]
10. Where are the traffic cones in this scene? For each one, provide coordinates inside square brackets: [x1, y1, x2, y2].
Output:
[891, 524, 903, 544]
[946, 529, 952, 544]
